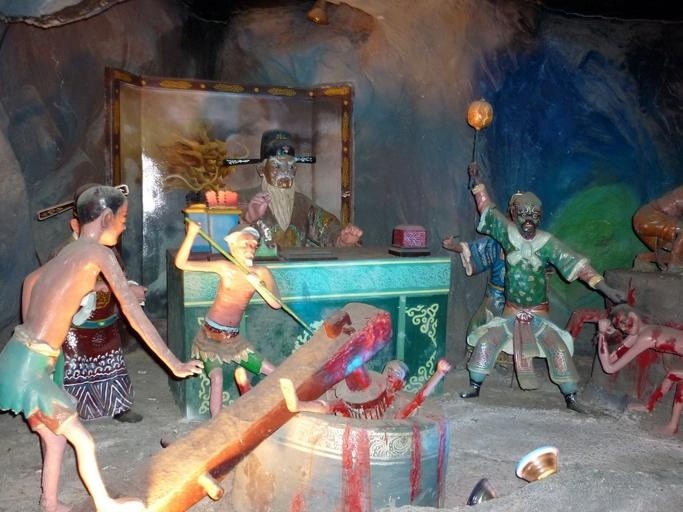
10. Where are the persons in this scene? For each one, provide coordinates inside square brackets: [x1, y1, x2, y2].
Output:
[458, 162, 627, 414]
[442, 189, 542, 393]
[279, 358, 454, 419]
[0, 182, 206, 512]
[36, 185, 147, 422]
[174, 227, 280, 420]
[593, 304, 683, 434]
[224, 131, 362, 250]
[630, 185, 682, 274]
